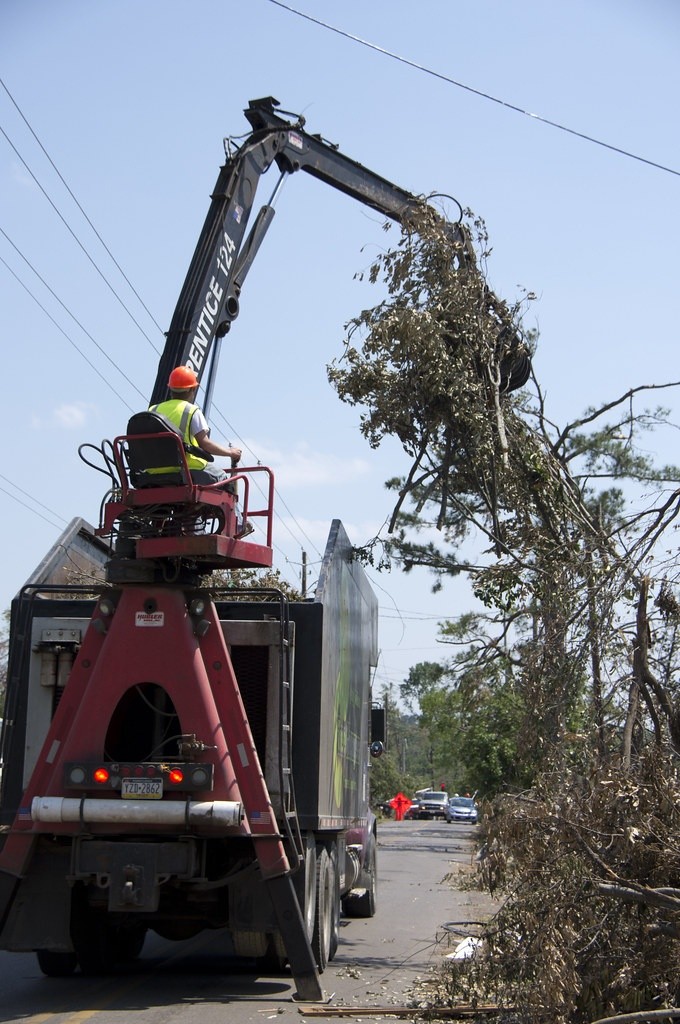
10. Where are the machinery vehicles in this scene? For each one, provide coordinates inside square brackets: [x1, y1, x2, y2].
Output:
[2, 514, 385, 975]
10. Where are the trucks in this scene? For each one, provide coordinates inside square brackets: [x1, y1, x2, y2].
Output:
[5, 85, 537, 1003]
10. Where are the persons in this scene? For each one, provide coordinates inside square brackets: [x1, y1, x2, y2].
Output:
[129, 366, 253, 538]
[439, 782, 470, 797]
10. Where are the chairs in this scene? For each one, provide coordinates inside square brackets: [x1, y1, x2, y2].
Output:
[126, 411, 218, 488]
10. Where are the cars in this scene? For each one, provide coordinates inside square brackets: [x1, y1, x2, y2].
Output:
[445, 796, 479, 825]
[376, 795, 419, 820]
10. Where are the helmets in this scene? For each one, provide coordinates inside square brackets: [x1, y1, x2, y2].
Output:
[167, 366, 198, 388]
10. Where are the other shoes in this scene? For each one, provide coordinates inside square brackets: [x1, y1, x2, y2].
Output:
[237, 524, 251, 533]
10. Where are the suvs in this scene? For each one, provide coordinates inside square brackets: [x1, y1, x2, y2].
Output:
[417, 789, 449, 820]
